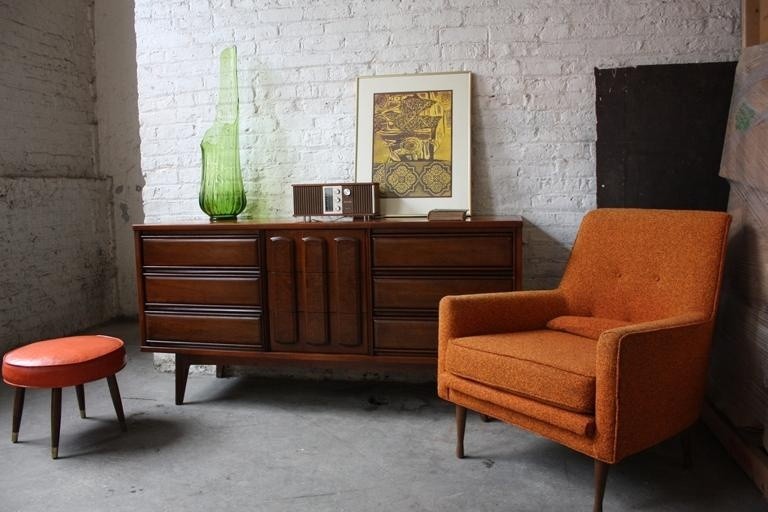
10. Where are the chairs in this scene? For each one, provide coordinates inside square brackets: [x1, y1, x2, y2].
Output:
[435, 206, 732, 512]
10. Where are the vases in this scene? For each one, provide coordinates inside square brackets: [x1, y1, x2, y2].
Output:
[199, 46, 248, 221]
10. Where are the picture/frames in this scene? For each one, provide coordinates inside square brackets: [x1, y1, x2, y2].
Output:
[354, 70, 474, 216]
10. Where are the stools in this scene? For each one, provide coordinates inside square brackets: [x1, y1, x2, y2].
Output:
[0, 336, 130, 458]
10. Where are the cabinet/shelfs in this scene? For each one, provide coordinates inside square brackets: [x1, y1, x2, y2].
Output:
[131, 218, 530, 411]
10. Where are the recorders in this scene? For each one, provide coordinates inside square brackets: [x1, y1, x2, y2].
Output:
[292, 183, 380, 221]
